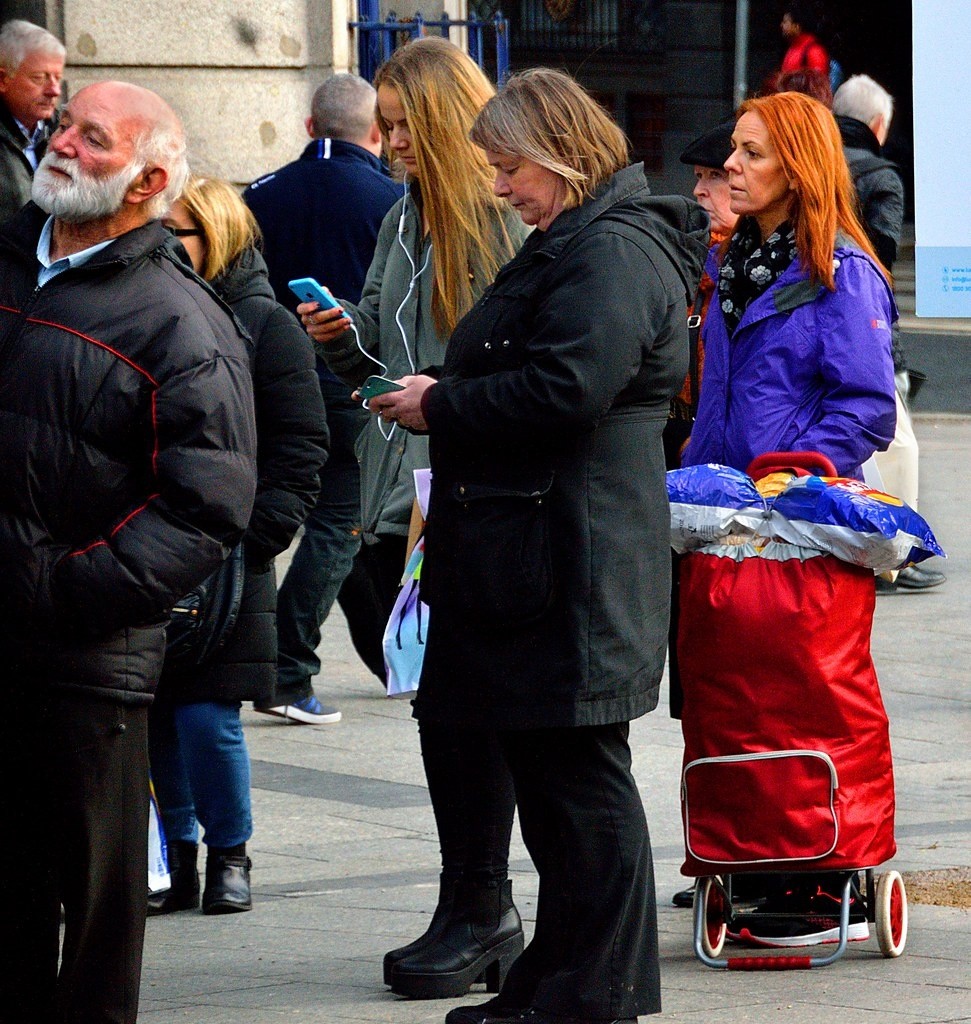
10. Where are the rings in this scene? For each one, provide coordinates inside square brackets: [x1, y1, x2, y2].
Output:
[306, 314, 317, 325]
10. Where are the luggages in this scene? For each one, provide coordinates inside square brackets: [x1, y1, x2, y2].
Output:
[681, 450, 910, 972]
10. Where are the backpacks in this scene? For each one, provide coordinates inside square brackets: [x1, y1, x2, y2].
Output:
[800, 40, 841, 95]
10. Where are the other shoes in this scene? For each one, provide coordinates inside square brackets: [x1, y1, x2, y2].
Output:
[444, 995, 639, 1024]
[673, 884, 696, 909]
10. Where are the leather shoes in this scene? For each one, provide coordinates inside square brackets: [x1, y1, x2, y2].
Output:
[876, 564, 946, 591]
[149, 847, 251, 915]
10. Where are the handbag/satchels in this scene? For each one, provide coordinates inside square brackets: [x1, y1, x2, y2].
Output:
[382, 468, 431, 696]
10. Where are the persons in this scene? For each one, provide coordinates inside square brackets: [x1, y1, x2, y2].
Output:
[830, 74, 946, 591]
[777, 10, 830, 88]
[241, 73, 407, 724]
[355, 69, 711, 1024]
[682, 92, 898, 946]
[0, 19, 67, 217]
[661, 118, 744, 910]
[143, 173, 330, 914]
[297, 36, 538, 997]
[0, 82, 257, 1024]
[776, 69, 833, 114]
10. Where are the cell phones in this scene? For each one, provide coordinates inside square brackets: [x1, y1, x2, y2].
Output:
[289, 276, 353, 324]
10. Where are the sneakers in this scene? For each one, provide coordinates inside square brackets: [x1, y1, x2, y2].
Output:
[723, 874, 871, 949]
[256, 695, 343, 724]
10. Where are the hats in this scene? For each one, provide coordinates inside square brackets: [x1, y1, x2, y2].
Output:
[680, 120, 739, 174]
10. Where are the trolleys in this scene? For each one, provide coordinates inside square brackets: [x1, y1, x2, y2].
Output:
[694, 451, 908, 968]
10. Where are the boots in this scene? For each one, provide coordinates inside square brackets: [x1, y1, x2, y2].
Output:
[383, 875, 524, 997]
